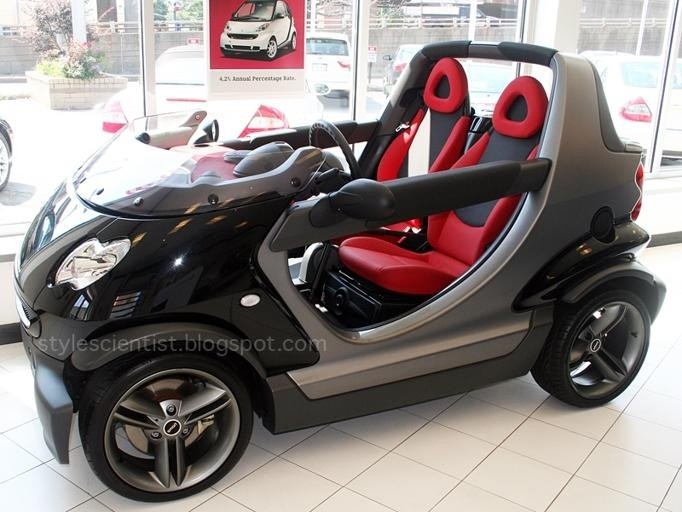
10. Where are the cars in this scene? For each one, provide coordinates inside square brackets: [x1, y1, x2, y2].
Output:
[0, 31, 352, 192]
[220, 0, 298, 61]
[383, 45, 682, 165]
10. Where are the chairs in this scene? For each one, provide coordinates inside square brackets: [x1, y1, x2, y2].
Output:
[339, 75, 547, 295]
[290, 57, 470, 245]
